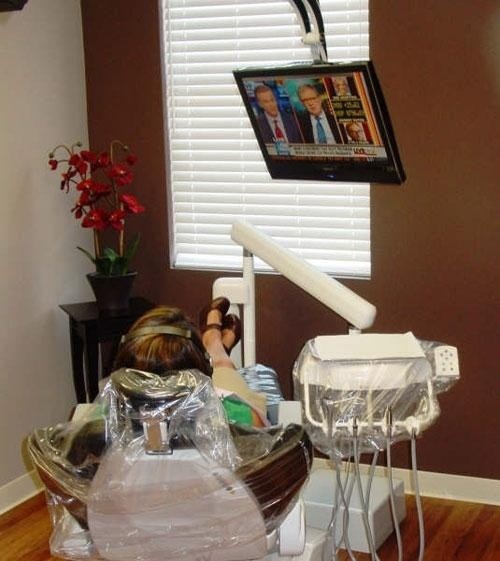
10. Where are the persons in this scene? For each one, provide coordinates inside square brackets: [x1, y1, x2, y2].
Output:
[297, 84, 344, 145]
[111, 296, 267, 429]
[250, 85, 303, 143]
[342, 120, 365, 143]
[332, 77, 351, 96]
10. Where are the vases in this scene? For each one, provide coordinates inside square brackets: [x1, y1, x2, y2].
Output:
[87, 272, 134, 316]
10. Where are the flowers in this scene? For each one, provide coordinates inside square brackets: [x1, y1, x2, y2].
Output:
[44, 138, 148, 277]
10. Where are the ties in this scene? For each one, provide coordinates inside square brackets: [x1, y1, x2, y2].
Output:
[314, 116, 327, 144]
[273, 120, 283, 139]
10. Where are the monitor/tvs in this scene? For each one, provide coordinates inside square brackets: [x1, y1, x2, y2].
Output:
[233, 61, 406, 183]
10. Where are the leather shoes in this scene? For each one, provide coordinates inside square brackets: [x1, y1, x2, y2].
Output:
[199, 297, 241, 357]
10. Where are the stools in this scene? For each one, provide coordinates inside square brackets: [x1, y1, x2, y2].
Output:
[59, 297, 157, 406]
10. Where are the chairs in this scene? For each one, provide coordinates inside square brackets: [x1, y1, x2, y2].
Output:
[21, 305, 312, 561]
